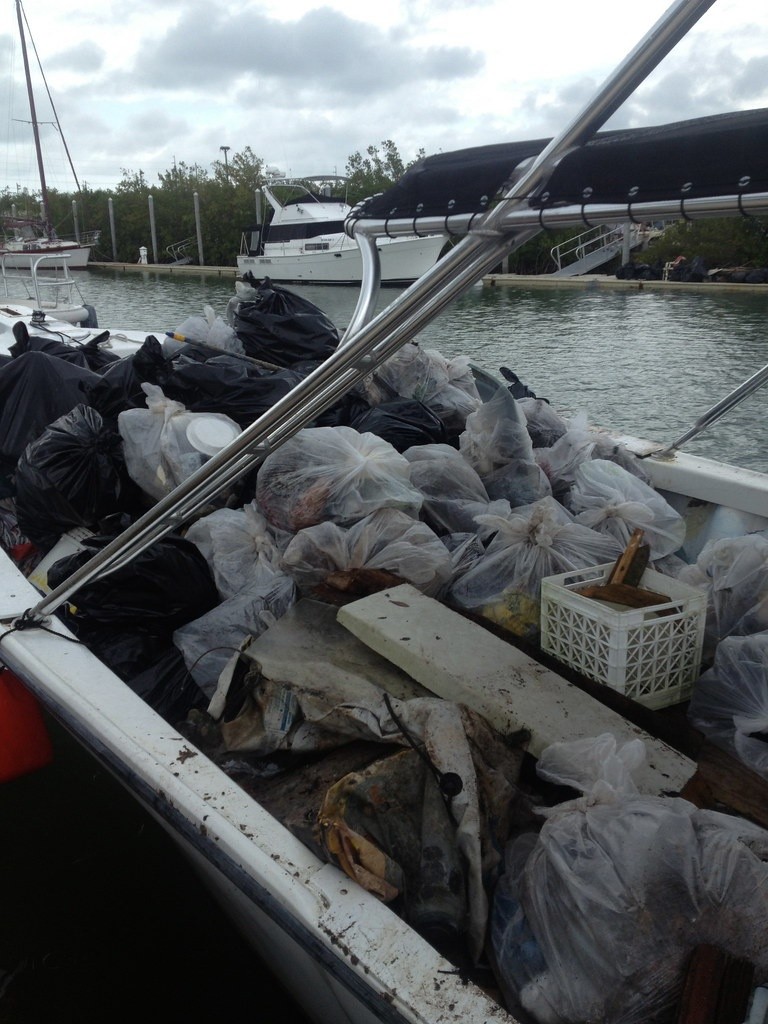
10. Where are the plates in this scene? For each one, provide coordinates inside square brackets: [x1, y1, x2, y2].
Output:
[186, 417, 238, 458]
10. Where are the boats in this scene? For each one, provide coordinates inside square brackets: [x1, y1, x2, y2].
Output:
[237, 181, 447, 286]
[1, 279, 768, 1024]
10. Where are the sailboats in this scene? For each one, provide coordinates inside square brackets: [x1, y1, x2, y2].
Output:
[0, 0, 90, 271]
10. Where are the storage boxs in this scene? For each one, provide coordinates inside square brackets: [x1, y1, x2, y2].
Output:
[541, 563, 708, 711]
